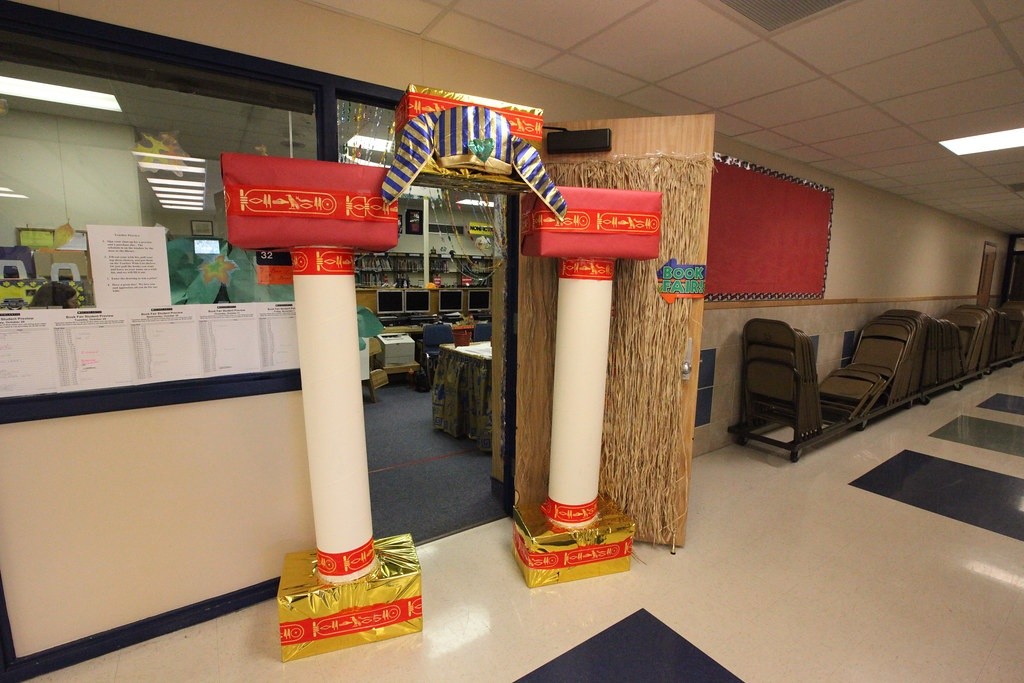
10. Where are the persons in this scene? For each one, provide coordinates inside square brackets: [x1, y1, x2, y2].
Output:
[27, 281, 78, 308]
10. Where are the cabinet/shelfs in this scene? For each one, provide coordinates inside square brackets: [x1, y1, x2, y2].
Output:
[358, 336, 391, 402]
[353, 248, 495, 290]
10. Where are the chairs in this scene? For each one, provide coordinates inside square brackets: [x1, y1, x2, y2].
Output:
[0, 259, 28, 279]
[50, 262, 81, 283]
[474, 323, 492, 344]
[418, 324, 454, 389]
[739, 300, 1024, 445]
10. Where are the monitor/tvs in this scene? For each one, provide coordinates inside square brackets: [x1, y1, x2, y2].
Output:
[193, 240, 220, 254]
[468, 290, 491, 313]
[405, 289, 431, 318]
[376, 290, 405, 319]
[439, 290, 463, 312]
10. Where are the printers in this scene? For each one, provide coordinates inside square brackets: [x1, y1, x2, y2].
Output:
[375, 332, 415, 366]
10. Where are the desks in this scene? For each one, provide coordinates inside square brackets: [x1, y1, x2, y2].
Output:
[382, 323, 476, 334]
[432, 341, 492, 452]
[0, 279, 87, 310]
